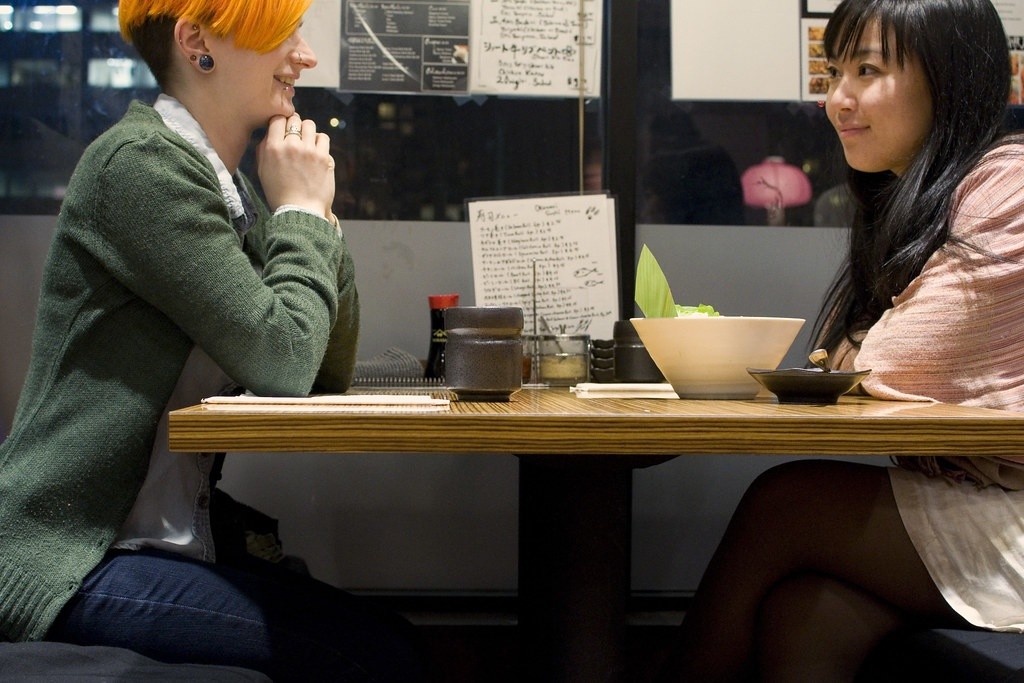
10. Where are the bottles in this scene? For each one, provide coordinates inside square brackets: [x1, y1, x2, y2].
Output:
[423, 295, 459, 379]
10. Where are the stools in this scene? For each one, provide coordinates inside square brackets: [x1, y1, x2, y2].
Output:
[0, 636, 275, 682]
[885, 620, 1024, 683]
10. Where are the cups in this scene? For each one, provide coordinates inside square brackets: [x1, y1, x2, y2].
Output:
[523, 334, 588, 387]
[444, 307, 524, 401]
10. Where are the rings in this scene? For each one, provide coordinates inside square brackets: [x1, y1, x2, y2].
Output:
[284, 124, 303, 138]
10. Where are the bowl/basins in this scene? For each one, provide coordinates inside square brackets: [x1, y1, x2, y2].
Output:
[591, 339, 614, 382]
[629, 317, 806, 400]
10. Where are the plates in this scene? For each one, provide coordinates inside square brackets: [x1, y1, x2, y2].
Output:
[747, 366, 872, 406]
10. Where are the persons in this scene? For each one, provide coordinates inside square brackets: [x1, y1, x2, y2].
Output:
[1, 2, 414, 682]
[654, 0, 1024, 683]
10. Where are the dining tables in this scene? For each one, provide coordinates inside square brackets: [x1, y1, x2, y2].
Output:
[167, 367, 1024, 683]
